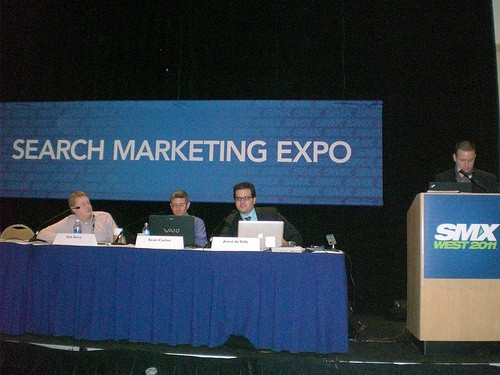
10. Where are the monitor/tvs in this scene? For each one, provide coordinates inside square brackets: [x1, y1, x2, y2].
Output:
[428, 182, 474, 193]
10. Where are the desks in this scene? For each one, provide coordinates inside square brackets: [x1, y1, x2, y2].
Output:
[0, 238, 349, 354]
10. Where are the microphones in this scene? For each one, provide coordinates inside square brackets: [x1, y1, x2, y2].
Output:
[110, 210, 164, 245]
[29, 206, 80, 242]
[459, 169, 490, 193]
[203, 207, 237, 249]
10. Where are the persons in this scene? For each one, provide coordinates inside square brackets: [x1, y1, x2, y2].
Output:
[37, 191, 118, 242]
[433, 141, 500, 193]
[170, 190, 208, 247]
[208, 182, 304, 248]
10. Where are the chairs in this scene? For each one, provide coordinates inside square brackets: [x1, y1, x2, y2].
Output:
[0, 224, 35, 241]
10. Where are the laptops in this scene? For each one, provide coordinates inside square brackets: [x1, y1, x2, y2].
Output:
[149, 214, 199, 247]
[237, 220, 284, 250]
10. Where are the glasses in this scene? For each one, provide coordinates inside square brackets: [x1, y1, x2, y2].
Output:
[235, 197, 252, 201]
[170, 203, 186, 209]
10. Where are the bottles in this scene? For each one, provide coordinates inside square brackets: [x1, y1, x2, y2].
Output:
[142, 222, 150, 235]
[73, 220, 82, 233]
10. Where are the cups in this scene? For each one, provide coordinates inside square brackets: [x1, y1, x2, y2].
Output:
[112, 228, 122, 243]
[282, 242, 295, 247]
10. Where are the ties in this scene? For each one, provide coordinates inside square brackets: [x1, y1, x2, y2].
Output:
[245, 217, 251, 221]
[463, 175, 469, 183]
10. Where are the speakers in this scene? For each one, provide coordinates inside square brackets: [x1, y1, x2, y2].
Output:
[326, 234, 339, 252]
